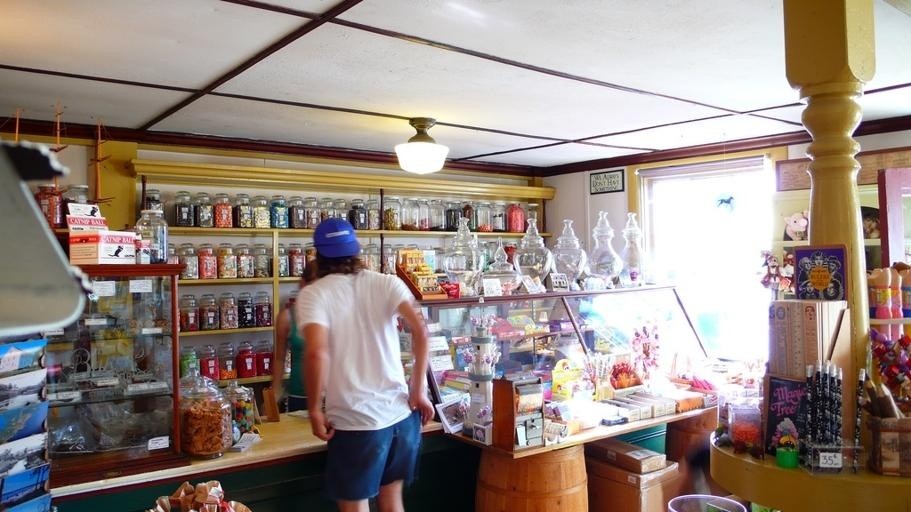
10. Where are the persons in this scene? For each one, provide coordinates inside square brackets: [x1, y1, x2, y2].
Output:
[798, 250, 842, 299]
[289, 216, 436, 511]
[269, 260, 319, 413]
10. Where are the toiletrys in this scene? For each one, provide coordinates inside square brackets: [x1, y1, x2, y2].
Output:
[719, 401, 732, 429]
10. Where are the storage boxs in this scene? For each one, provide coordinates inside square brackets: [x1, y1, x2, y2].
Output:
[586, 455, 682, 512]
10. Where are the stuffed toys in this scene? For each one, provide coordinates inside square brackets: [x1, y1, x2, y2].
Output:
[760, 247, 782, 299]
[782, 209, 809, 242]
[862, 216, 882, 238]
[781, 253, 795, 294]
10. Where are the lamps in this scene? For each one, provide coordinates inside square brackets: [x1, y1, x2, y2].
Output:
[392, 116, 450, 176]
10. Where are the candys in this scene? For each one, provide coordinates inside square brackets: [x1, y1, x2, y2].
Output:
[34, 184, 79, 230]
[136, 182, 539, 279]
[397, 251, 728, 445]
[135, 289, 298, 434]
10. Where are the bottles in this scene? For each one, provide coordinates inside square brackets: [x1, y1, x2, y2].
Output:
[68, 184, 88, 203]
[619, 212, 648, 281]
[132, 186, 541, 460]
[587, 211, 625, 282]
[550, 218, 585, 290]
[515, 218, 553, 290]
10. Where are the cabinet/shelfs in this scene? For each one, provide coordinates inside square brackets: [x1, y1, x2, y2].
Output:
[47, 264, 187, 474]
[277, 227, 554, 380]
[138, 221, 277, 392]
[777, 237, 911, 327]
[394, 284, 709, 422]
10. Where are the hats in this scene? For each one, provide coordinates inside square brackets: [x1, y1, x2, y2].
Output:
[313, 218, 360, 258]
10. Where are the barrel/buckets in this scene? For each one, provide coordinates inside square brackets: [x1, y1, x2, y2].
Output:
[666, 408, 717, 462]
[475, 445, 590, 512]
[667, 494, 747, 512]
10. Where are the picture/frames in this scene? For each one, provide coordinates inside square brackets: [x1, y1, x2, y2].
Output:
[589, 168, 625, 195]
